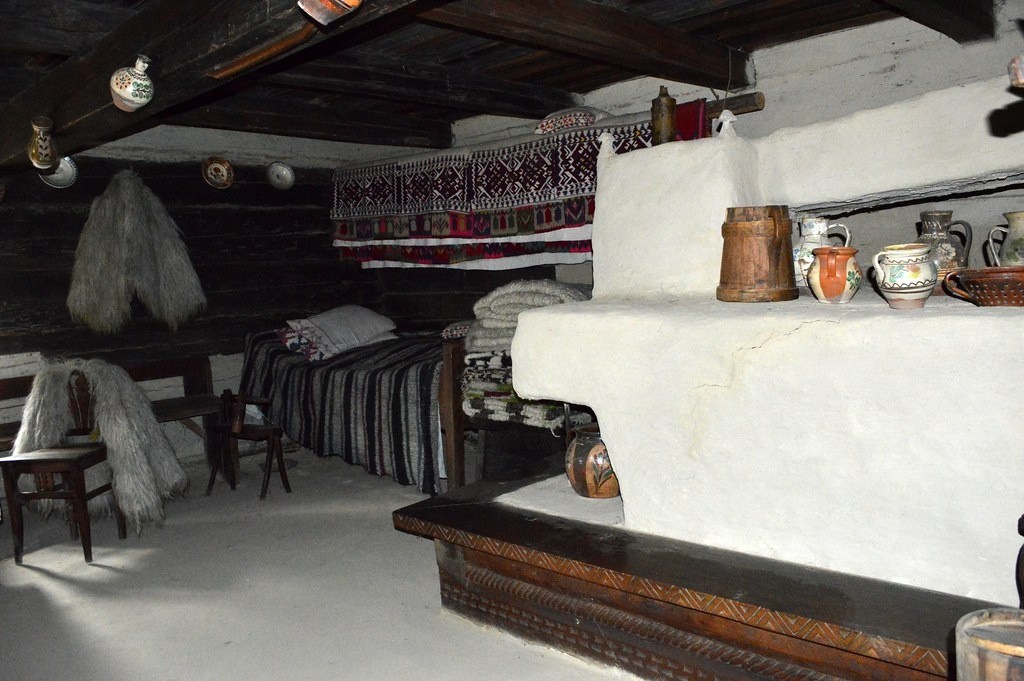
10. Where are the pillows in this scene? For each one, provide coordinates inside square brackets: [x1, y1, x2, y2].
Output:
[533, 106, 617, 135]
[274, 327, 400, 362]
[286, 305, 396, 358]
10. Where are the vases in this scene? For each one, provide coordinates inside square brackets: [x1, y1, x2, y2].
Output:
[25, 115, 59, 176]
[110, 54, 154, 113]
[650, 85, 674, 144]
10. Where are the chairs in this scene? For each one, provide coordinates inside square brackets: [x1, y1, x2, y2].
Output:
[0, 357, 186, 567]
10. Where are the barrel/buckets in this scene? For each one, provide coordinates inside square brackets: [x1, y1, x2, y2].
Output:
[955, 608, 1024, 681]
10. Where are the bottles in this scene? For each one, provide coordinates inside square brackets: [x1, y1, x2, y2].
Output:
[871, 242, 938, 309]
[109, 54, 154, 113]
[565, 423, 619, 498]
[27, 116, 58, 169]
[651, 85, 676, 147]
[808, 247, 863, 305]
[297, 0, 363, 26]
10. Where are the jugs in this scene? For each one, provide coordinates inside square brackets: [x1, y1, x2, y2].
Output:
[792, 216, 851, 286]
[916, 211, 973, 296]
[989, 211, 1024, 267]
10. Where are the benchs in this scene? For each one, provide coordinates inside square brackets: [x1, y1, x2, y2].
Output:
[123, 349, 234, 500]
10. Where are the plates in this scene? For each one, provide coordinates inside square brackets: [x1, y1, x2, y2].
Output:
[202, 156, 235, 190]
[265, 161, 295, 190]
[37, 153, 78, 188]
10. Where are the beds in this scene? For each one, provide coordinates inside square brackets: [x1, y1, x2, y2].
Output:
[241, 325, 466, 499]
